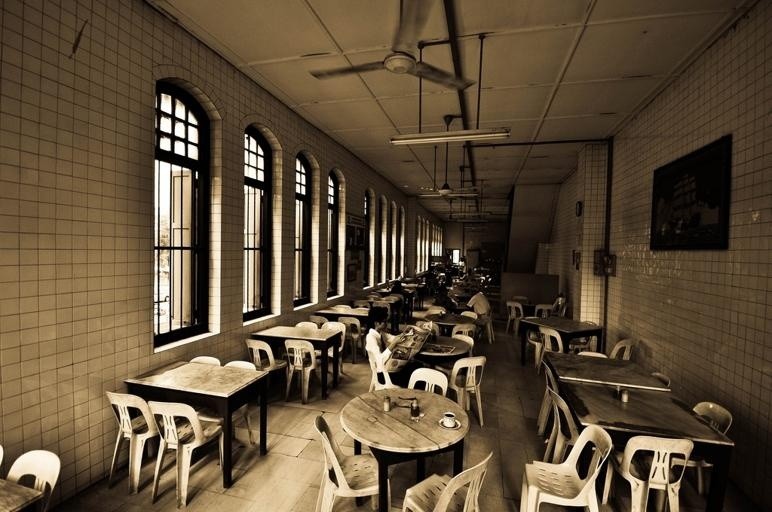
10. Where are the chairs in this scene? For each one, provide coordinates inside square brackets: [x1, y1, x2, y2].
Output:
[147, 401, 225, 508]
[314, 415, 392, 512]
[616, 436, 695, 510]
[446, 355, 487, 428]
[402, 450, 494, 512]
[519, 424, 614, 511]
[542, 385, 580, 466]
[366, 351, 401, 392]
[106, 391, 159, 495]
[434, 335, 475, 379]
[578, 351, 609, 360]
[222, 361, 257, 444]
[6, 449, 61, 512]
[339, 316, 366, 363]
[650, 371, 672, 388]
[282, 340, 318, 404]
[245, 339, 288, 406]
[309, 260, 497, 344]
[537, 361, 560, 436]
[189, 356, 221, 367]
[296, 322, 318, 330]
[408, 368, 448, 399]
[0, 445, 3, 467]
[314, 321, 346, 379]
[609, 338, 637, 402]
[506, 295, 595, 376]
[668, 402, 733, 494]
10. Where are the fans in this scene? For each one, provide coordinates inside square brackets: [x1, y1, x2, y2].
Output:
[309, 0, 477, 92]
[417, 114, 479, 198]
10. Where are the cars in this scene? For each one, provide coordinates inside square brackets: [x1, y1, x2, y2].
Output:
[154, 267, 170, 302]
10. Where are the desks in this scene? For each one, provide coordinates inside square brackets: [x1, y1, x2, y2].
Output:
[123, 361, 269, 489]
[545, 351, 733, 511]
[314, 306, 371, 318]
[519, 317, 604, 368]
[252, 326, 342, 400]
[407, 337, 470, 357]
[0, 480, 43, 512]
[425, 313, 475, 334]
[340, 388, 470, 511]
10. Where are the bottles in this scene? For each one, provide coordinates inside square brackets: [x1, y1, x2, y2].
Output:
[542, 313, 546, 319]
[383, 394, 391, 412]
[614, 385, 630, 404]
[410, 400, 420, 421]
[387, 285, 389, 290]
[439, 311, 443, 318]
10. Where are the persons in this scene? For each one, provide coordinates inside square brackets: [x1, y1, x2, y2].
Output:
[391, 259, 491, 340]
[364, 307, 424, 390]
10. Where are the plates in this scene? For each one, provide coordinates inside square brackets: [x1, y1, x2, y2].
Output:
[438, 418, 462, 431]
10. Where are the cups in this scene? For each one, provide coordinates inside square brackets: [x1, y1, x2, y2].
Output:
[443, 412, 456, 428]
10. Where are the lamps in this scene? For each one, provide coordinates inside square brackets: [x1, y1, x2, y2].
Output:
[389, 33, 512, 145]
